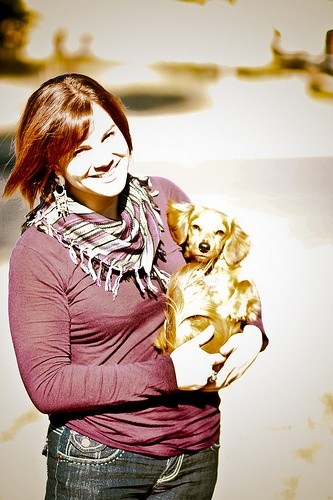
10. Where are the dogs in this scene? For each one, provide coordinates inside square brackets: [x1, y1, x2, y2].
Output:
[152, 199, 261, 372]
